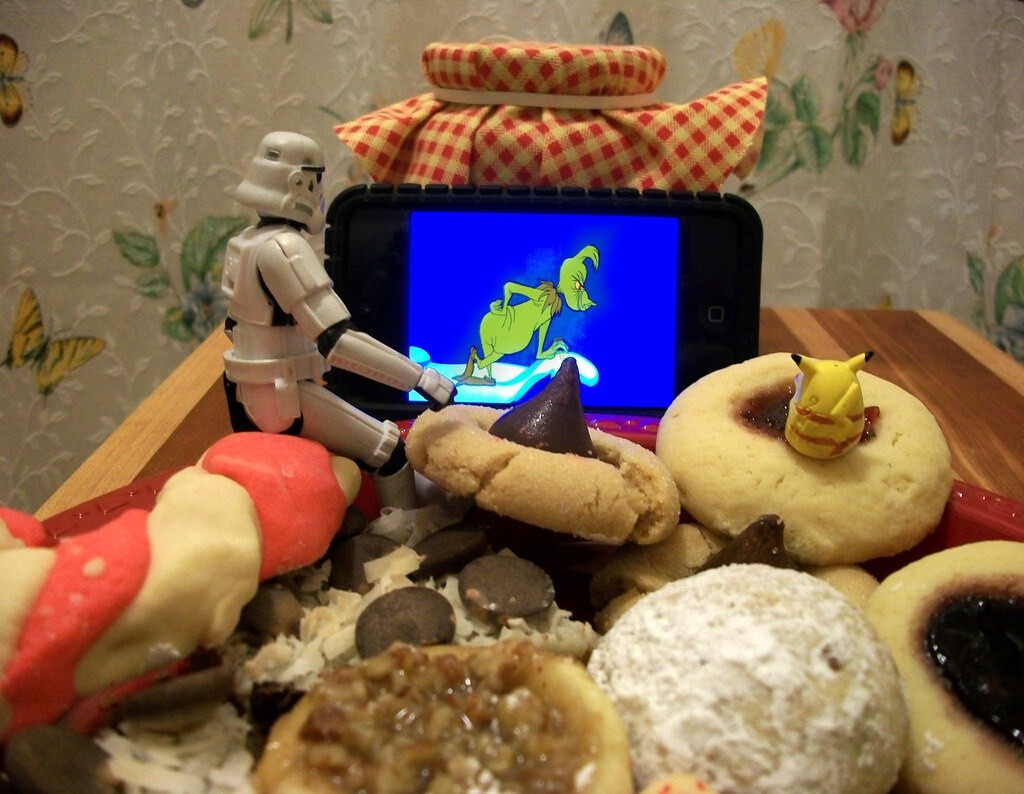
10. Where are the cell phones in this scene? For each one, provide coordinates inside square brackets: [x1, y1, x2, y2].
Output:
[324, 182, 762, 422]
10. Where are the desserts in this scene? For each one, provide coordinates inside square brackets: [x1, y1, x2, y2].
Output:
[0, 351, 1024, 794]
